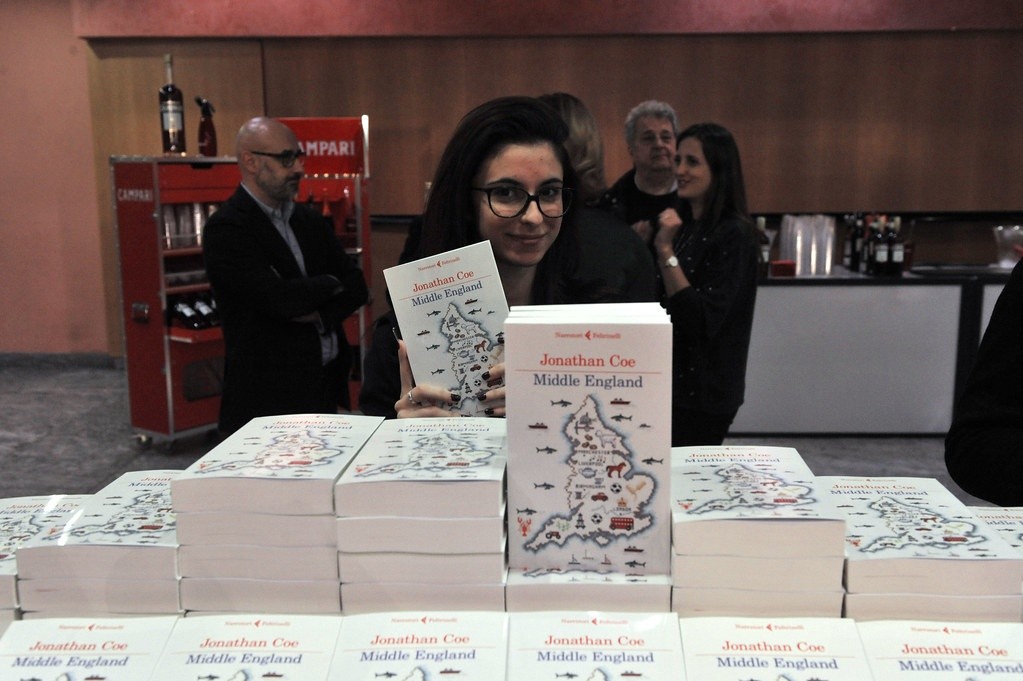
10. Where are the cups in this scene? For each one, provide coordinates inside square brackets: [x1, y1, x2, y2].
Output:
[162, 204, 222, 248]
[780, 214, 834, 277]
[994, 224, 1023, 269]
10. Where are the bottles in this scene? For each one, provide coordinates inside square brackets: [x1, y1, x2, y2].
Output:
[298, 187, 356, 237]
[844, 214, 905, 279]
[172, 300, 218, 329]
[755, 216, 777, 262]
[158, 53, 187, 157]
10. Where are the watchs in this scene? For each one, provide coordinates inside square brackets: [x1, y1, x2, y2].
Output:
[660, 256, 679, 269]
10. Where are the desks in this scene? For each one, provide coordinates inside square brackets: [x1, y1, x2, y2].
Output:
[728, 267, 1012, 438]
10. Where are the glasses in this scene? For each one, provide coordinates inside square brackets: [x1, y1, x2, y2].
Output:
[475, 186, 575, 218]
[252, 151, 306, 168]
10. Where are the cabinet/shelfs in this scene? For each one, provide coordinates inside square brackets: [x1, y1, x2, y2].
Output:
[109, 153, 372, 454]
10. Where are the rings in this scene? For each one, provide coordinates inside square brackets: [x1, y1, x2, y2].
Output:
[664, 215, 669, 219]
[409, 391, 420, 405]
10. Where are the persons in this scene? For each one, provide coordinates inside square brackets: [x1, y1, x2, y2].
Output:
[541, 91, 760, 447]
[358, 95, 585, 419]
[945, 258, 1023, 507]
[203, 117, 368, 435]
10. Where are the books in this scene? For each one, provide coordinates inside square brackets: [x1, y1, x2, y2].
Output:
[382, 238, 509, 417]
[0, 303, 1022, 681]
